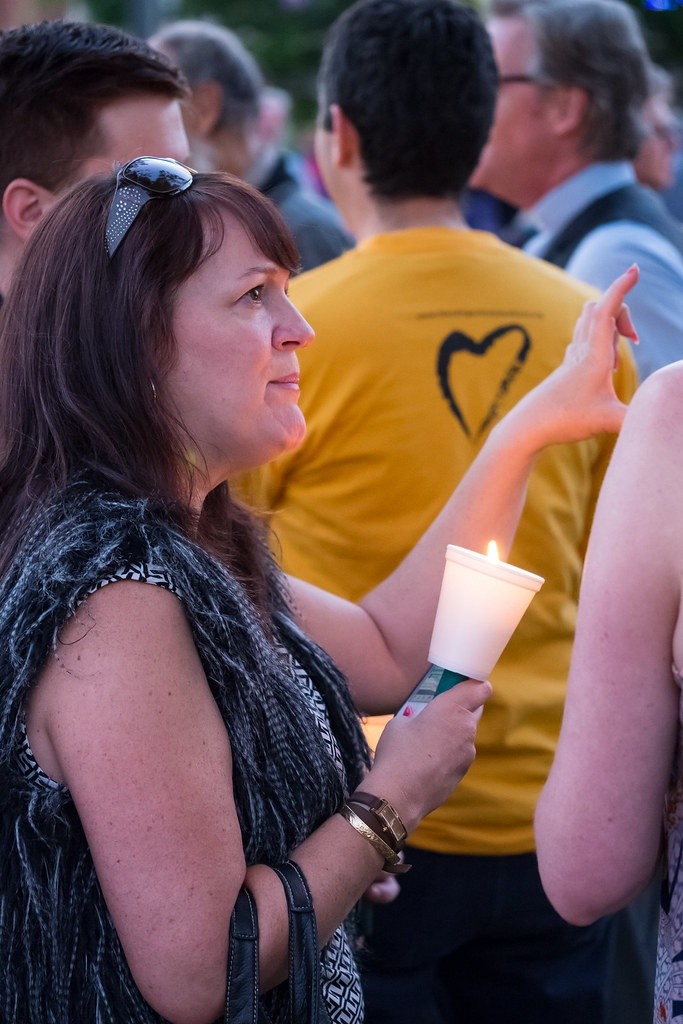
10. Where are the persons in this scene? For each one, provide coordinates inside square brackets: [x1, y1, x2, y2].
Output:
[0, 153, 641, 1024]
[0, 0, 682, 387]
[215, 0, 637, 1024]
[536, 363, 682, 1024]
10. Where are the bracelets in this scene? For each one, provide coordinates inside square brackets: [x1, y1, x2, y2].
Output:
[339, 803, 413, 874]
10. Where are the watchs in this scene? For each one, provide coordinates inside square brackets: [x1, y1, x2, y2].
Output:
[348, 791, 408, 854]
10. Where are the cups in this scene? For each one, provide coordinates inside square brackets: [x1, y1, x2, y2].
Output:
[426, 545, 544, 683]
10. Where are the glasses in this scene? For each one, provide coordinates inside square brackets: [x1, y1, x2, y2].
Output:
[499, 74, 542, 88]
[104, 155, 197, 262]
